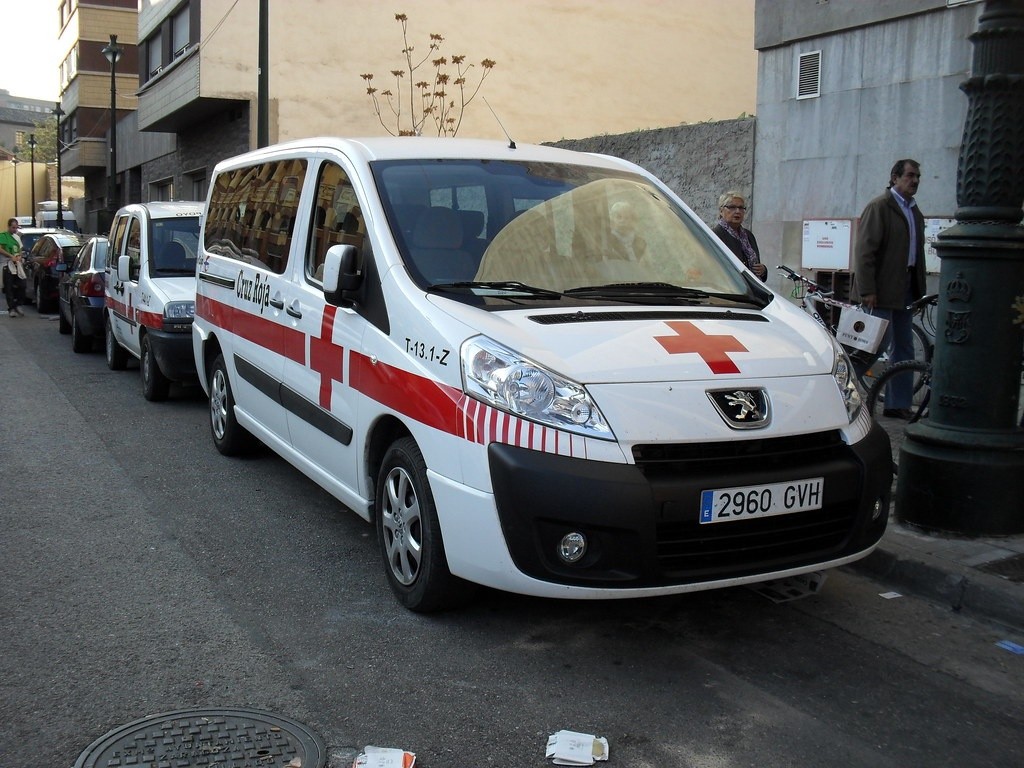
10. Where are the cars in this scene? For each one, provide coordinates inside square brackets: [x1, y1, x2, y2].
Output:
[21, 234, 96, 314]
[56, 237, 195, 354]
[17, 227, 57, 266]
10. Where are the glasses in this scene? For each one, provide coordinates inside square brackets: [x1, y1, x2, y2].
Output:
[721, 204, 746, 211]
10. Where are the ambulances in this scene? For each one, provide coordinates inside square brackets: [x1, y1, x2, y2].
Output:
[101, 201, 248, 401]
[192, 138, 895, 616]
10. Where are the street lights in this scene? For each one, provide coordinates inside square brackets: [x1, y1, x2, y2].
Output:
[10, 155, 20, 217]
[50, 102, 67, 229]
[26, 134, 38, 226]
[102, 34, 125, 228]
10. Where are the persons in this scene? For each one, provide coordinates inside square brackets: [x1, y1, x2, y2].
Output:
[607, 201, 648, 264]
[208, 199, 362, 235]
[0, 218, 27, 317]
[848, 159, 926, 421]
[711, 190, 768, 283]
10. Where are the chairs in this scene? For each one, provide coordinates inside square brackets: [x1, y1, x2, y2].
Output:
[456, 209, 489, 268]
[392, 203, 429, 249]
[410, 204, 478, 283]
[157, 241, 186, 265]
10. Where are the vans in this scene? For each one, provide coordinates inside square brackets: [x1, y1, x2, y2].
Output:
[36, 211, 81, 238]
[10, 216, 36, 229]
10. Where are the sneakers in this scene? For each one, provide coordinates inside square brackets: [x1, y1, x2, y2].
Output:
[9, 309, 17, 317]
[16, 306, 24, 316]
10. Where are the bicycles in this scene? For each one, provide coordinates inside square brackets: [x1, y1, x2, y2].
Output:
[776, 264, 934, 401]
[865, 293, 938, 474]
